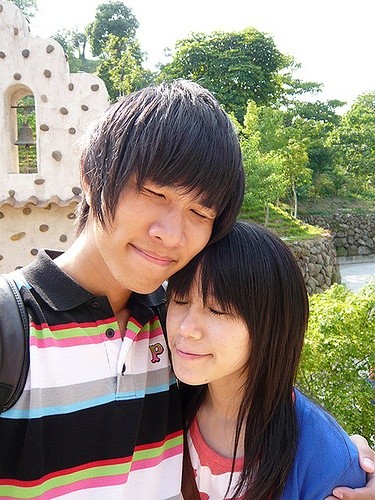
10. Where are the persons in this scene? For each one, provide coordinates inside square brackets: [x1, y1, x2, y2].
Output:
[0, 78, 374, 500]
[165, 222, 370, 500]
